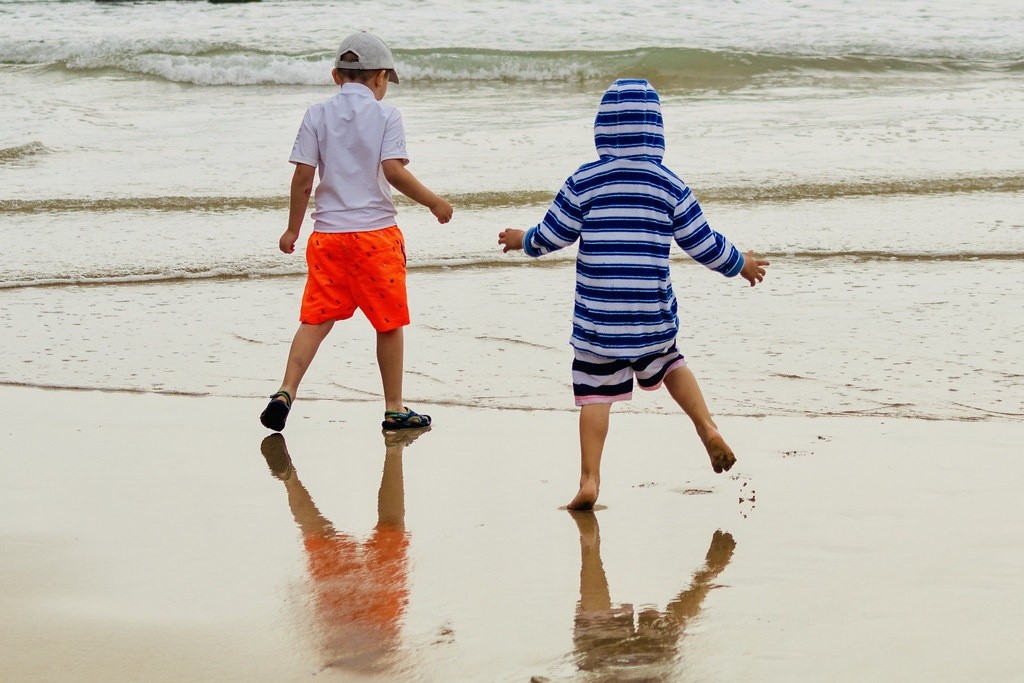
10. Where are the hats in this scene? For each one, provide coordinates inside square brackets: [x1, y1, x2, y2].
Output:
[335, 31, 400, 85]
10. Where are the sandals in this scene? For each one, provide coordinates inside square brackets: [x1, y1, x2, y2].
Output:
[260, 391, 291, 432]
[382, 407, 432, 430]
[383, 425, 432, 447]
[261, 432, 294, 476]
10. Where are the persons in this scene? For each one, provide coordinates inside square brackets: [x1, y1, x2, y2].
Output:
[259, 31, 453, 431]
[498, 79, 770, 510]
